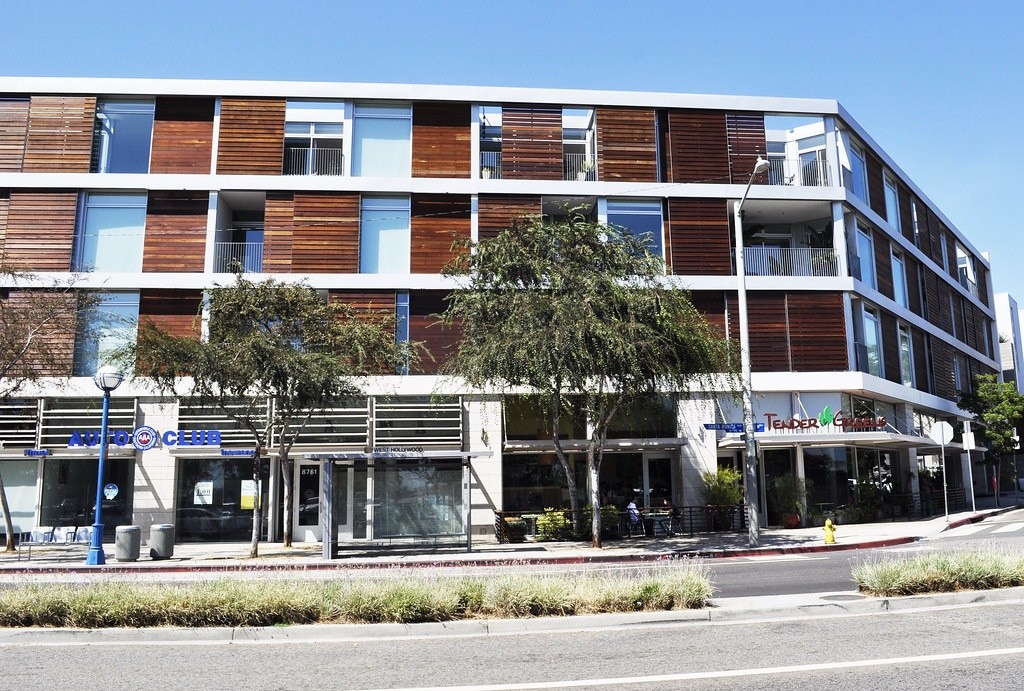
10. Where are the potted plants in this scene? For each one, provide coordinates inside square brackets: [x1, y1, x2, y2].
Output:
[808, 506, 834, 526]
[576, 160, 595, 181]
[851, 475, 880, 522]
[701, 463, 745, 531]
[835, 507, 864, 524]
[775, 472, 815, 529]
[482, 167, 491, 179]
[883, 492, 907, 517]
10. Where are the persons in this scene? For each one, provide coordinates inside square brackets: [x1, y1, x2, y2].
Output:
[627, 496, 651, 540]
[921, 470, 936, 518]
[659, 496, 681, 535]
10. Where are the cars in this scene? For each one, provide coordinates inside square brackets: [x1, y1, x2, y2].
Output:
[176, 503, 252, 539]
[55, 497, 121, 520]
[299, 496, 320, 518]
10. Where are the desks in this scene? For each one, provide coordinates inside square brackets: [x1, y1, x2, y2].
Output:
[642, 512, 668, 537]
[815, 502, 835, 509]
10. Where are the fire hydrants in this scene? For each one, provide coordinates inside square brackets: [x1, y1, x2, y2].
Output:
[821, 518, 837, 544]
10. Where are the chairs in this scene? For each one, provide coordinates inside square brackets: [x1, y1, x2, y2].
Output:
[672, 512, 684, 535]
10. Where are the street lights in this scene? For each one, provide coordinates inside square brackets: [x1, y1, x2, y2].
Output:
[86, 365, 125, 565]
[734, 156, 771, 548]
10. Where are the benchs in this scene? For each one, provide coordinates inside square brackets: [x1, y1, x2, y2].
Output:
[18, 526, 92, 561]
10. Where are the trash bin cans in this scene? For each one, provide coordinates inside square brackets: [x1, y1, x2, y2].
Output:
[150, 524, 175, 560]
[115, 526, 141, 562]
